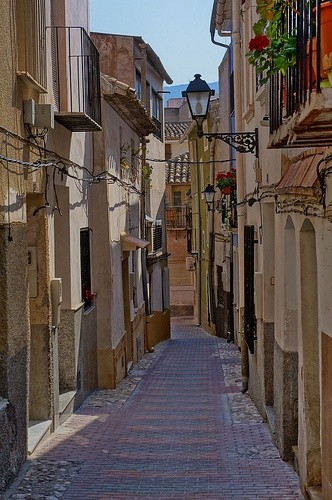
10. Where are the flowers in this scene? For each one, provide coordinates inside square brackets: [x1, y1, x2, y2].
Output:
[214, 168, 236, 189]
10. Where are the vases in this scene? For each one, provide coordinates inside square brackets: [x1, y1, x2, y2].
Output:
[306, 38, 327, 79]
[223, 186, 231, 195]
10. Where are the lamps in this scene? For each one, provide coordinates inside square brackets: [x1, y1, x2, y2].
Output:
[203, 184, 226, 212]
[181, 73, 260, 158]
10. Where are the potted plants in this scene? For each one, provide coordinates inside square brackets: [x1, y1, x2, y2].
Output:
[306, 0, 332, 72]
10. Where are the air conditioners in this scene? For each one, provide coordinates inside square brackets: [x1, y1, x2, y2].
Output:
[186, 257, 196, 271]
